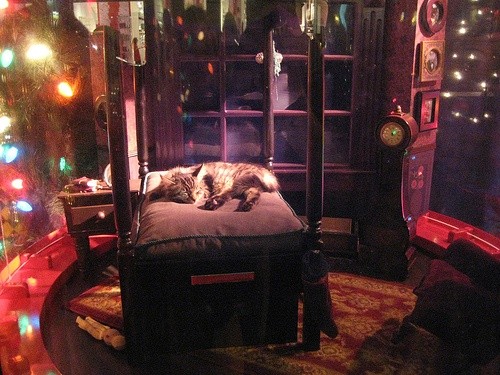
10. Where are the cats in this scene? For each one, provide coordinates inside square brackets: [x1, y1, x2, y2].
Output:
[148, 159, 281, 212]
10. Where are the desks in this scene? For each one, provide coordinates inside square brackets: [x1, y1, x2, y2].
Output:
[57, 179, 143, 281]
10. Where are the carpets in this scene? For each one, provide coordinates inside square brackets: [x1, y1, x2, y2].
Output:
[61, 269, 441, 375]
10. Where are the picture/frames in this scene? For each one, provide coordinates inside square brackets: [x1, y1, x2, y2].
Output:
[417, 90, 440, 131]
